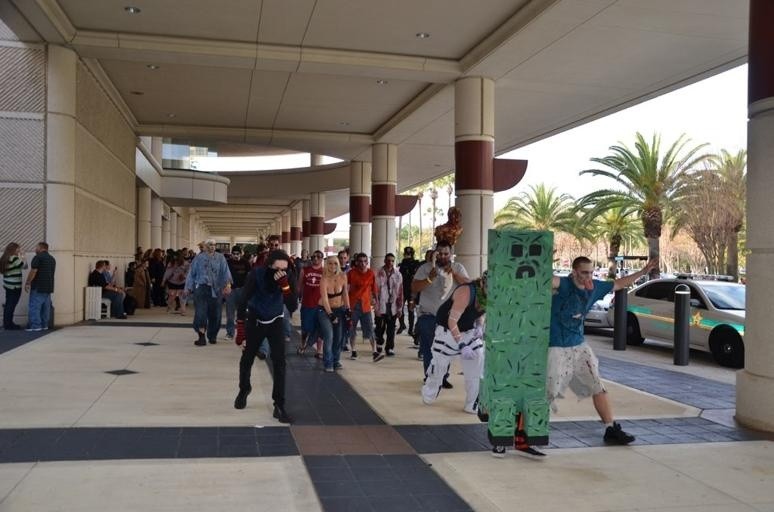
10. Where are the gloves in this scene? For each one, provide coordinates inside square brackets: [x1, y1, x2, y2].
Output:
[458, 341, 477, 361]
[275, 275, 293, 299]
[236, 319, 247, 346]
[345, 308, 352, 320]
[327, 312, 336, 323]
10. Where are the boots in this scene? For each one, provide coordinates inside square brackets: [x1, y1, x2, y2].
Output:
[2, 303, 21, 330]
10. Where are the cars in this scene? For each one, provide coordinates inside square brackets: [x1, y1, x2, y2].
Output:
[607, 276, 746, 367]
[554, 273, 612, 329]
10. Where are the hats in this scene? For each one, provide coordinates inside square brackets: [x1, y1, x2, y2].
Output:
[404, 247, 415, 255]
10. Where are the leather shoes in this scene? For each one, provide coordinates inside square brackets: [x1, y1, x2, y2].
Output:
[273, 403, 296, 423]
[234, 383, 252, 408]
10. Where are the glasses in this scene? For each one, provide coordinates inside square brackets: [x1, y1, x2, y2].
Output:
[271, 245, 279, 246]
[233, 252, 240, 256]
[312, 255, 324, 259]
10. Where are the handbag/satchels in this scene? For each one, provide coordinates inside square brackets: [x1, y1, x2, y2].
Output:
[123, 295, 137, 314]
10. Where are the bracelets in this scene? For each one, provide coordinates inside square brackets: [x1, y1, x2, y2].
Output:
[426, 276, 432, 284]
[226, 283, 231, 288]
[115, 288, 117, 292]
[282, 286, 290, 290]
[410, 301, 414, 304]
[458, 342, 466, 350]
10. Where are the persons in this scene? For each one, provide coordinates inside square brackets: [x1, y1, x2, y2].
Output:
[254, 234, 297, 360]
[127, 246, 182, 316]
[411, 249, 435, 347]
[231, 249, 298, 424]
[396, 246, 420, 337]
[101, 260, 127, 315]
[242, 243, 254, 267]
[0, 241, 29, 331]
[315, 256, 352, 373]
[252, 243, 268, 265]
[546, 255, 661, 446]
[344, 252, 385, 363]
[223, 245, 253, 341]
[410, 238, 472, 388]
[288, 257, 298, 279]
[23, 242, 56, 332]
[296, 250, 325, 360]
[369, 252, 405, 356]
[89, 261, 126, 319]
[182, 238, 234, 346]
[294, 248, 311, 303]
[197, 241, 207, 254]
[351, 253, 359, 268]
[182, 247, 196, 314]
[420, 268, 489, 415]
[337, 250, 352, 352]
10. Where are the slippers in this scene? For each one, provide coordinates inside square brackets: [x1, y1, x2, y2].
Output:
[314, 352, 323, 360]
[297, 343, 308, 356]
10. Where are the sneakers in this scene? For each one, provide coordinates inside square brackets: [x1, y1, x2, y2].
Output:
[24, 327, 48, 331]
[334, 363, 344, 369]
[443, 378, 452, 388]
[386, 347, 395, 358]
[373, 352, 384, 362]
[417, 350, 425, 362]
[224, 334, 234, 340]
[350, 351, 359, 360]
[603, 421, 635, 445]
[396, 323, 406, 334]
[194, 332, 206, 345]
[408, 329, 415, 337]
[514, 446, 545, 460]
[375, 343, 383, 354]
[324, 368, 336, 375]
[491, 444, 508, 461]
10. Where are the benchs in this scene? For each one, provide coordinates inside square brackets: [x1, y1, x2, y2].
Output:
[85, 287, 112, 321]
